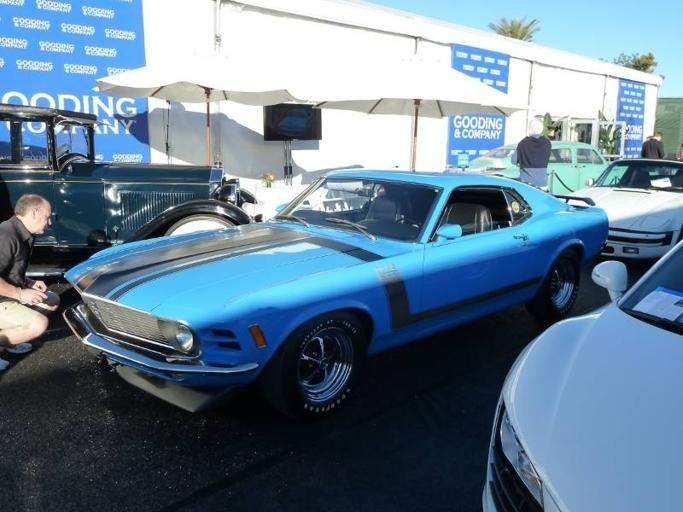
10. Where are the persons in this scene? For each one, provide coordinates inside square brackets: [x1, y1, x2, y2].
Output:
[640, 132, 666, 159]
[510, 118, 551, 193]
[0, 194, 61, 374]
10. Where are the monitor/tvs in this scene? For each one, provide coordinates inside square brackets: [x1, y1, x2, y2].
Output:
[264, 104, 322, 141]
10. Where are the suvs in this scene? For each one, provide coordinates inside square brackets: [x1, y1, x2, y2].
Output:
[0, 100, 263, 279]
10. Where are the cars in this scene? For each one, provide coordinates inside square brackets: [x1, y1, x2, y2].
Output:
[564, 158, 683, 260]
[61, 168, 611, 423]
[480, 245, 683, 511]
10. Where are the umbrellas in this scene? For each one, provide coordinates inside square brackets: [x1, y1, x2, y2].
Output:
[290, 47, 525, 172]
[93, 45, 308, 166]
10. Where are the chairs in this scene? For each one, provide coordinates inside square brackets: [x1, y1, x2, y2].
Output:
[632, 170, 682, 190]
[363, 194, 492, 240]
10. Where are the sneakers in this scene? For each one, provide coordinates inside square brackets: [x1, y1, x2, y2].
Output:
[0, 342, 32, 371]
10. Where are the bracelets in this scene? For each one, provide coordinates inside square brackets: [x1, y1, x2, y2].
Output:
[17, 286, 24, 305]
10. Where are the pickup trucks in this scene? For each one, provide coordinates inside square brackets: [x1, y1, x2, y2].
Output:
[466, 140, 660, 201]
